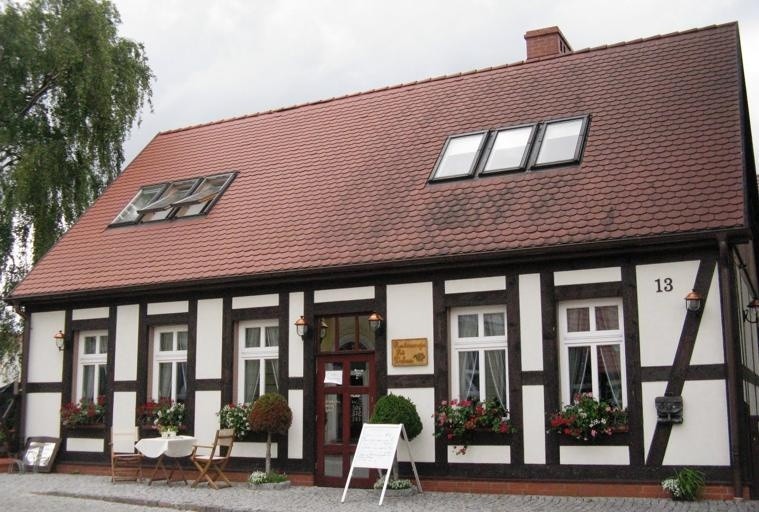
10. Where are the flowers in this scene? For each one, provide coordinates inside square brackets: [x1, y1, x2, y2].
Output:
[428, 395, 517, 456]
[373, 475, 413, 489]
[248, 469, 289, 485]
[743, 299, 759, 324]
[136, 397, 185, 434]
[60, 394, 106, 429]
[660, 470, 711, 503]
[543, 393, 629, 442]
[214, 402, 253, 442]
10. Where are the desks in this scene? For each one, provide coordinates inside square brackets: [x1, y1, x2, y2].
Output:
[140, 436, 196, 487]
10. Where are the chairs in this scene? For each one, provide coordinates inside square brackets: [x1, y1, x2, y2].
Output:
[189, 427, 236, 490]
[108, 426, 145, 485]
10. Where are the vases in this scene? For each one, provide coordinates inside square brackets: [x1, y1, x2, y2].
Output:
[160, 431, 177, 439]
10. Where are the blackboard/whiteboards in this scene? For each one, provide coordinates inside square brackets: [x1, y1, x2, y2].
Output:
[353, 424, 402, 469]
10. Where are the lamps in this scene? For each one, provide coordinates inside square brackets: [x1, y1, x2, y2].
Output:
[684, 289, 703, 318]
[368, 313, 385, 340]
[294, 316, 309, 341]
[53, 330, 65, 352]
